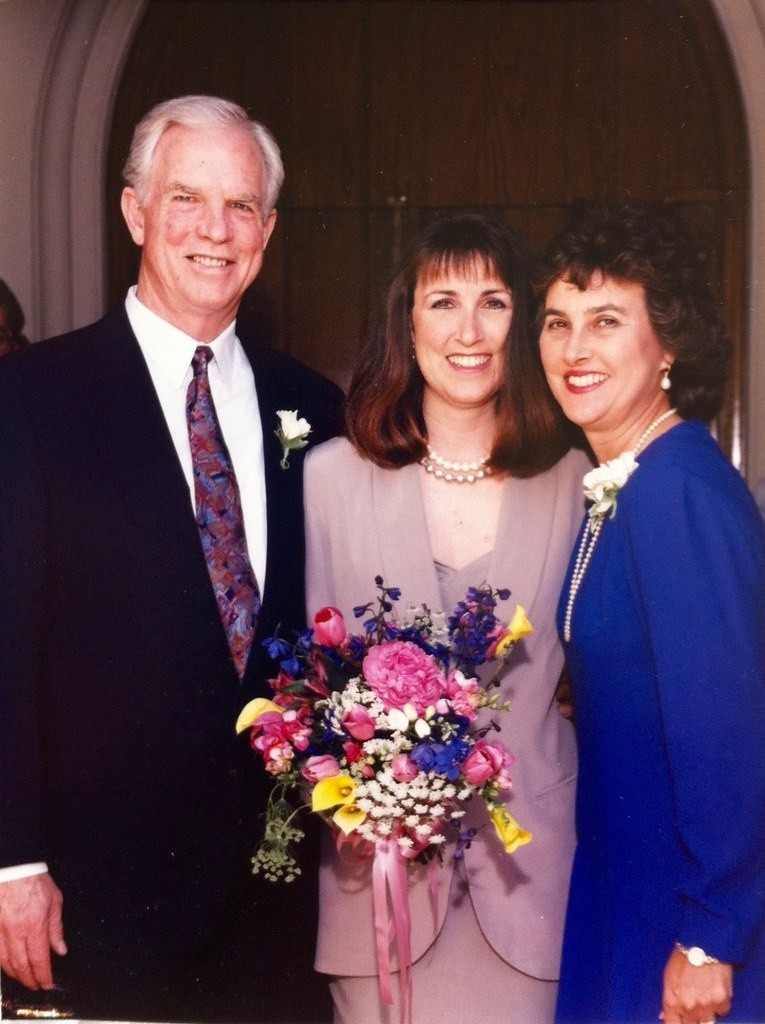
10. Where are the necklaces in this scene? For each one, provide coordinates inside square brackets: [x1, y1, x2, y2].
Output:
[418, 440, 496, 484]
[564, 409, 680, 640]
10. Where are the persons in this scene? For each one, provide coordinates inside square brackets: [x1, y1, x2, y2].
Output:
[0, 97, 576, 1024]
[529, 202, 765, 1024]
[301, 213, 591, 1024]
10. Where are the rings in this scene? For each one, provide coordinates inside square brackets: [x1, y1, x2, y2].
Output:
[701, 1020, 715, 1024]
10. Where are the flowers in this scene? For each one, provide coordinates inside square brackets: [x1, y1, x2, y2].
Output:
[273, 409, 314, 470]
[235, 573, 536, 888]
[582, 451, 641, 534]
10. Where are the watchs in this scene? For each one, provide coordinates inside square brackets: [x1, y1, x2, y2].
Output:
[674, 940, 719, 966]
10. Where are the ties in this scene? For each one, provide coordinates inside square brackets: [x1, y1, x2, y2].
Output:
[186, 346, 262, 688]
